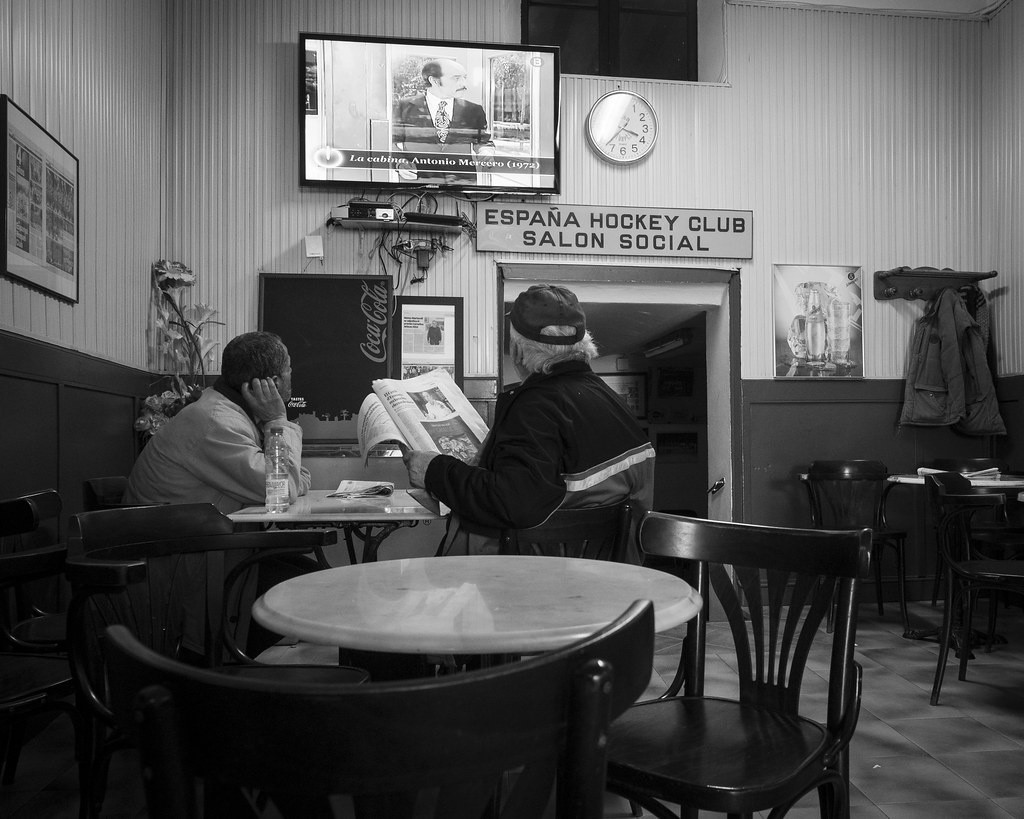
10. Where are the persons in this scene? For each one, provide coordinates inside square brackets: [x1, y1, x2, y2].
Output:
[427, 320, 442, 345]
[391, 58, 496, 185]
[403, 366, 454, 380]
[120, 329, 329, 677]
[337, 284, 656, 684]
[420, 390, 453, 419]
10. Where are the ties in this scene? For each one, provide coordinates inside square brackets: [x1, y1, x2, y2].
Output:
[434, 101, 452, 147]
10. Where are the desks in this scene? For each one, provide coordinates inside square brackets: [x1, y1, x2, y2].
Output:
[226, 485, 451, 566]
[252, 554, 706, 684]
[886, 468, 1024, 654]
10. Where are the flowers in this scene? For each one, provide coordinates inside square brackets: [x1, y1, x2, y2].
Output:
[133, 255, 225, 447]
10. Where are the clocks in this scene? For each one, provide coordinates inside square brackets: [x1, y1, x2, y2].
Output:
[586, 92, 659, 164]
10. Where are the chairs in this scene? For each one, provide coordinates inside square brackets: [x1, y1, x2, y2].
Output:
[931, 457, 1023, 652]
[0, 474, 873, 819]
[920, 472, 1024, 707]
[797, 458, 912, 633]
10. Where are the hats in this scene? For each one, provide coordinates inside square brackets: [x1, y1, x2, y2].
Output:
[504, 284, 585, 345]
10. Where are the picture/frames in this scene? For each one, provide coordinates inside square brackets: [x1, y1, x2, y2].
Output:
[0, 92, 82, 304]
[393, 296, 464, 395]
[256, 272, 393, 445]
[595, 372, 648, 420]
[771, 264, 866, 380]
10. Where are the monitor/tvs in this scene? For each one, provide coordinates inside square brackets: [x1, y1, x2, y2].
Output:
[297, 31, 561, 196]
